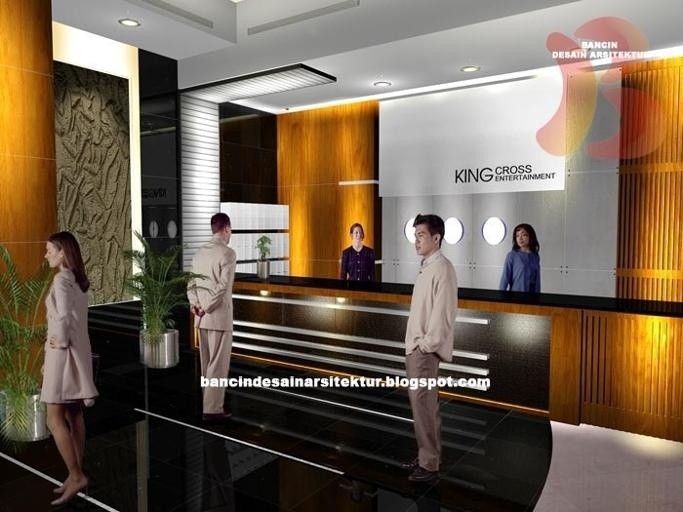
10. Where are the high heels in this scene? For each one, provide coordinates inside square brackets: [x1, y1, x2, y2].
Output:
[51, 471, 89, 505]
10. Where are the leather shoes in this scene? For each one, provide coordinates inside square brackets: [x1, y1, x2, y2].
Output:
[203, 413, 232, 421]
[399, 458, 443, 482]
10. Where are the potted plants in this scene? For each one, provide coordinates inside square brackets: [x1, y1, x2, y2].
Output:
[254, 235, 273, 279]
[120, 230, 215, 369]
[0, 243, 56, 453]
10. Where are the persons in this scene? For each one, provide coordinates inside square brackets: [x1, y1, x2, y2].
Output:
[39, 232, 99, 505]
[500, 224, 540, 293]
[340, 223, 375, 282]
[187, 213, 236, 420]
[400, 215, 458, 482]
